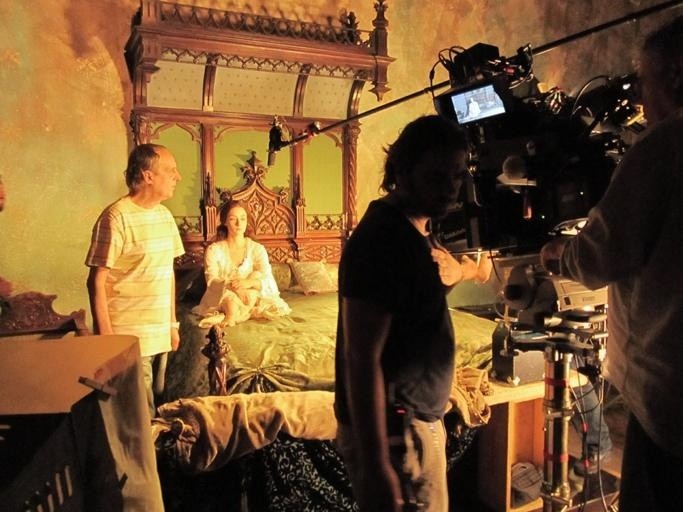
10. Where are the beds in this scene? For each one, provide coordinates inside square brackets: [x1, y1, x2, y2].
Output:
[163, 258, 591, 510]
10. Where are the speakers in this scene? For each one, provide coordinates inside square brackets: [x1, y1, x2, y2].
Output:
[493, 319, 545, 385]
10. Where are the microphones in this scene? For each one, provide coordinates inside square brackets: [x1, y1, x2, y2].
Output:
[268, 114, 278, 167]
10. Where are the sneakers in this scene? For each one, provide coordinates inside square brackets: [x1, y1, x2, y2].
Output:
[574, 446, 614, 476]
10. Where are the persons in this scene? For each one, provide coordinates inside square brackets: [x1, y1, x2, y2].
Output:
[451, 252, 496, 286]
[567, 384, 614, 478]
[191, 200, 293, 328]
[332, 114, 468, 512]
[468, 98, 480, 116]
[85, 143, 187, 424]
[538, 17, 683, 512]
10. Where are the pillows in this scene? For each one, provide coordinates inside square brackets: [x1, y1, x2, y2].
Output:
[270, 260, 339, 294]
